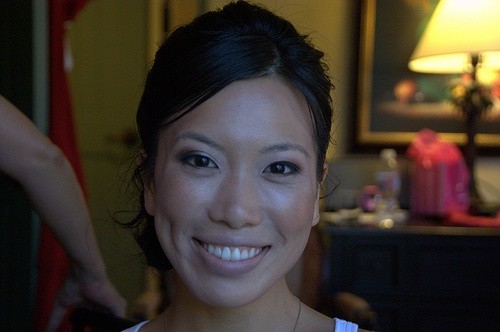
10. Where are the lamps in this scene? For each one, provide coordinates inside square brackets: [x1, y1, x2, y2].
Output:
[407, 0, 500, 218]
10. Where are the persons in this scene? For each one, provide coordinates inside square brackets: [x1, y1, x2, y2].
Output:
[119, 1, 374, 332]
[0, 96, 127, 332]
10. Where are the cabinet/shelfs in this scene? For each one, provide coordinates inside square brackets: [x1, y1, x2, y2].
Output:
[318, 220, 500, 332]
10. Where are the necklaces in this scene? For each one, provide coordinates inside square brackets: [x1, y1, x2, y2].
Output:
[292, 296, 302, 332]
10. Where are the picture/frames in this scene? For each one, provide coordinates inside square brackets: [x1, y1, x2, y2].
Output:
[347, 0, 500, 160]
